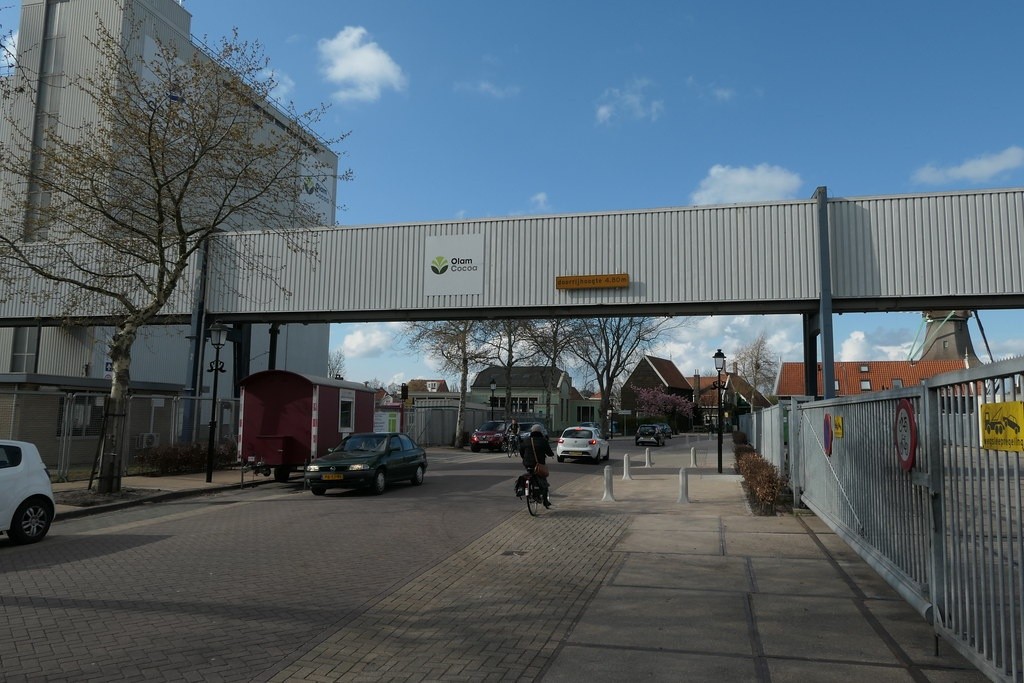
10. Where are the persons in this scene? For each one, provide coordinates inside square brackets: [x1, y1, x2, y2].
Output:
[505, 419, 520, 450]
[519, 425, 551, 506]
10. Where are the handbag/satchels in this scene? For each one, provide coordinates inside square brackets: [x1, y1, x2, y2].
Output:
[534, 463, 549, 477]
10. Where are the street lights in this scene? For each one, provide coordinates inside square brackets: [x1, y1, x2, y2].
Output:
[712, 348, 726, 474]
[487, 380, 499, 421]
[203, 319, 229, 484]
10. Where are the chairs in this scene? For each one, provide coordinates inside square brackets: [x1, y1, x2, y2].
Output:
[360, 441, 375, 451]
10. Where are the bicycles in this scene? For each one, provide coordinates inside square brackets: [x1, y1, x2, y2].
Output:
[520, 453, 557, 516]
[505, 433, 520, 458]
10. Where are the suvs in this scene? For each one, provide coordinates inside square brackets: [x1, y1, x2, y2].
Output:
[635, 425, 665, 446]
[469, 421, 511, 453]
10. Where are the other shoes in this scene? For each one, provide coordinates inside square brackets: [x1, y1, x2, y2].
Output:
[543, 501, 552, 506]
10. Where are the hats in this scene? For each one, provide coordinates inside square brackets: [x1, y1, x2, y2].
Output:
[531, 423, 544, 434]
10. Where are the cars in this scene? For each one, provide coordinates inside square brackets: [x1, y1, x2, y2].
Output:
[513, 422, 547, 442]
[555, 427, 609, 463]
[657, 423, 673, 438]
[0, 439, 57, 546]
[305, 432, 428, 495]
